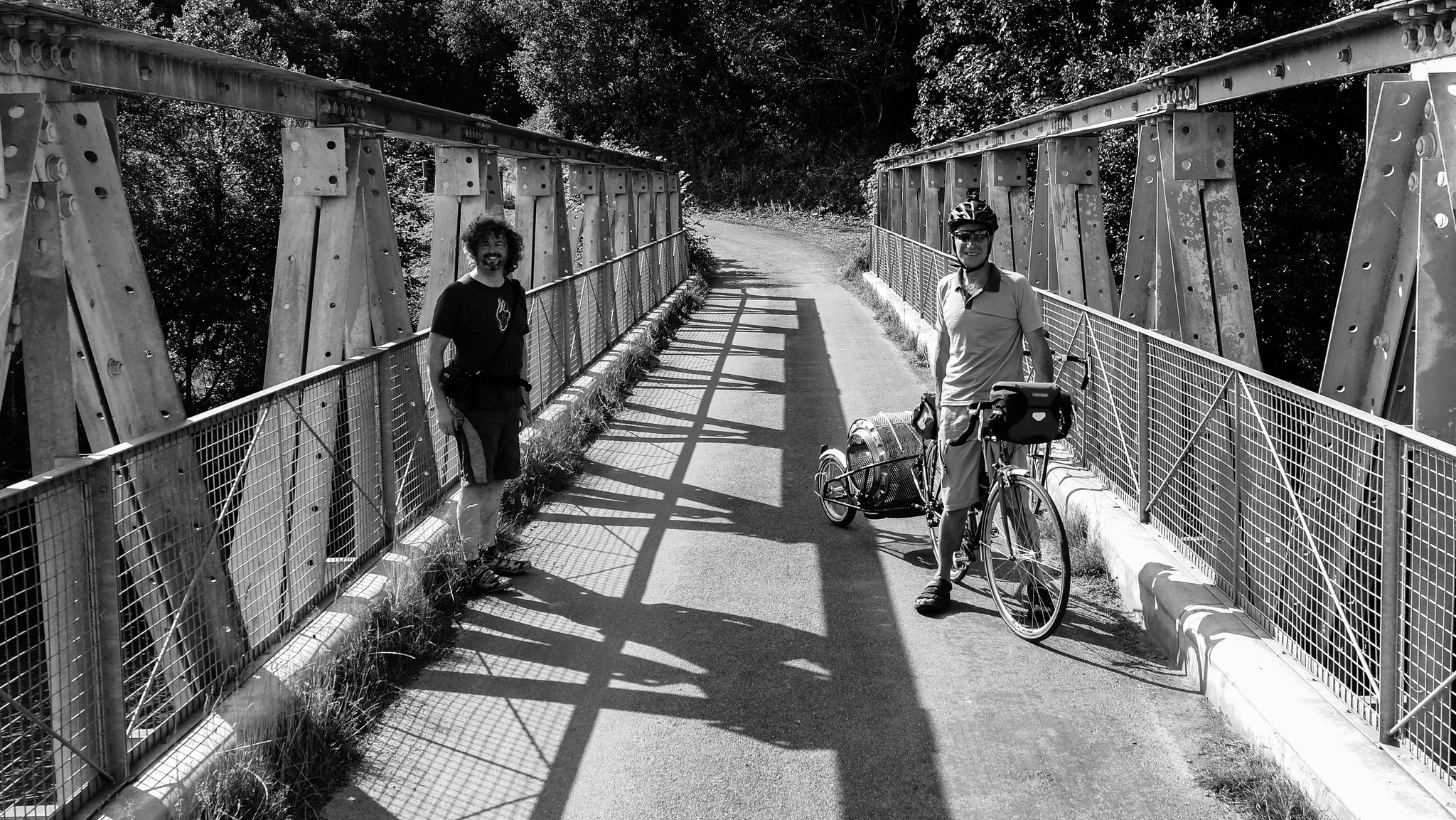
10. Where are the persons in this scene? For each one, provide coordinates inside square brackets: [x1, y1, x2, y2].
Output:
[424, 213, 533, 591]
[910, 200, 1064, 624]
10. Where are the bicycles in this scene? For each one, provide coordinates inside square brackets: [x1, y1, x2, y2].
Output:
[912, 379, 1078, 645]
[1021, 350, 1090, 517]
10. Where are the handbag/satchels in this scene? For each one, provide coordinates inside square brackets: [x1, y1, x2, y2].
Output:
[439, 366, 470, 399]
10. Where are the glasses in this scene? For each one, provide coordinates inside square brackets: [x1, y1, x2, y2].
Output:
[953, 229, 991, 240]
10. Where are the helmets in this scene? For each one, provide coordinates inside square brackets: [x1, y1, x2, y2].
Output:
[947, 200, 999, 230]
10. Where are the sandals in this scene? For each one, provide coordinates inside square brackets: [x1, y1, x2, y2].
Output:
[913, 578, 953, 611]
[1027, 582, 1055, 619]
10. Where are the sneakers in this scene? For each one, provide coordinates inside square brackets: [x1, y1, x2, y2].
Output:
[463, 547, 533, 591]
